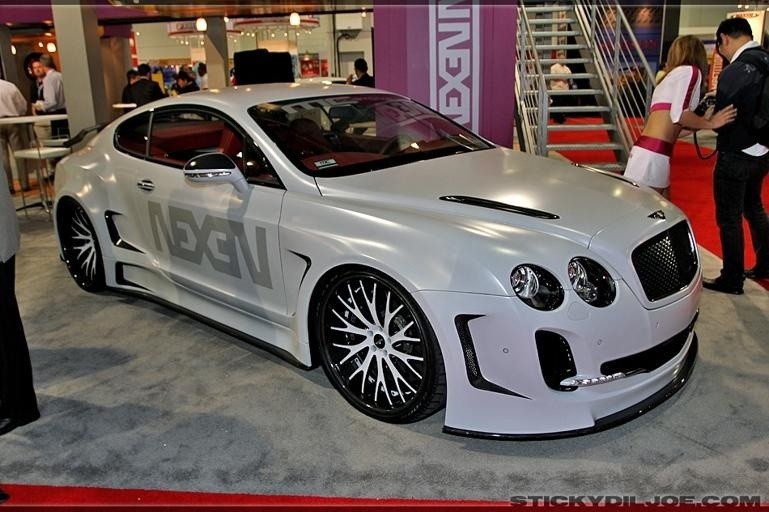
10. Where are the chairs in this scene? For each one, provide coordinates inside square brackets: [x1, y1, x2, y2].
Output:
[287, 115, 333, 159]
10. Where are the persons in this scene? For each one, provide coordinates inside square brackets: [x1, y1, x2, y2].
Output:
[551, 51, 575, 124]
[701, 18, 769, 295]
[34, 54, 71, 185]
[1, 79, 29, 195]
[25, 61, 57, 182]
[343, 58, 375, 88]
[120, 62, 208, 112]
[623, 35, 739, 196]
[1, 137, 40, 437]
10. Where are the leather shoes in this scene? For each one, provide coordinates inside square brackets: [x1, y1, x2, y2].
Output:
[744, 263, 769, 280]
[0, 416, 17, 435]
[702, 276, 744, 295]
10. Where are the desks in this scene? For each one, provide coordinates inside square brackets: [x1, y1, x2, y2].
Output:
[0, 110, 70, 223]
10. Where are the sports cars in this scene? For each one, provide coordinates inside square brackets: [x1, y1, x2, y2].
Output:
[52, 78, 706, 438]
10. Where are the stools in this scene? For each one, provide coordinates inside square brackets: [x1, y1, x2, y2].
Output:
[12, 146, 69, 214]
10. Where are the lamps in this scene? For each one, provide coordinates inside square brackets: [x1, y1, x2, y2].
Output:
[195, 14, 208, 33]
[289, 8, 301, 26]
